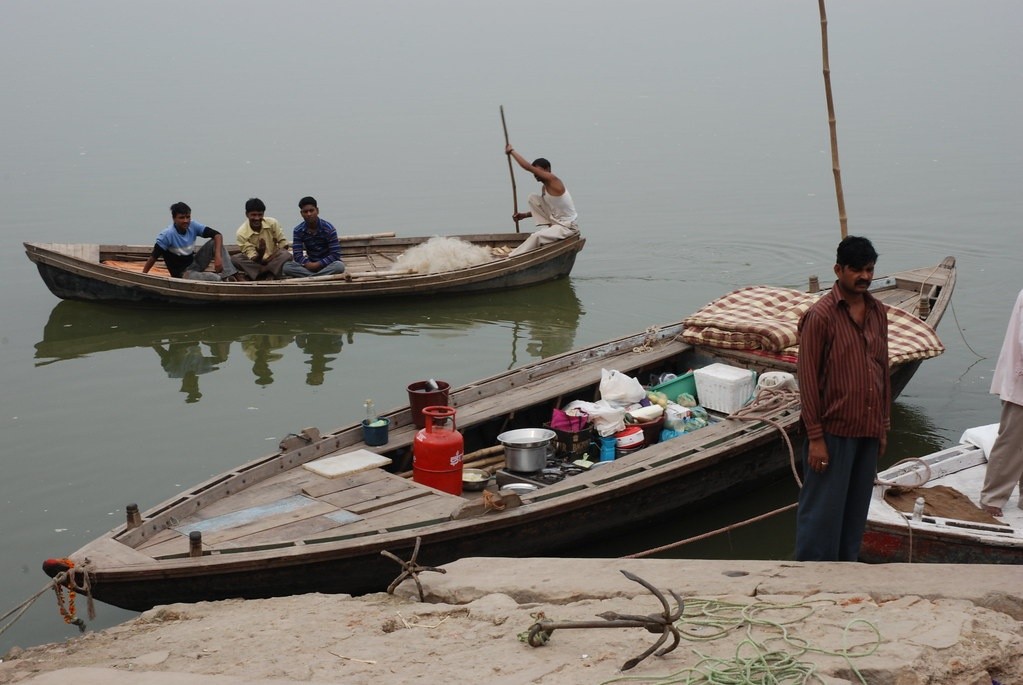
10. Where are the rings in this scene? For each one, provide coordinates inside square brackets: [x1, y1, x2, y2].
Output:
[821, 461, 827, 465]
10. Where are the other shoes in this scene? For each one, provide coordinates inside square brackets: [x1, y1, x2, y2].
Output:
[226, 276, 238, 281]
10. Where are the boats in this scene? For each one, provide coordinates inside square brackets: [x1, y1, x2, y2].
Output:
[21, 230, 589, 305]
[39, 256, 961, 614]
[856, 421, 1023, 567]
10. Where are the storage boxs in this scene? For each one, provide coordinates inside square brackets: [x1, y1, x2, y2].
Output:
[694, 363, 757, 414]
[649, 373, 694, 402]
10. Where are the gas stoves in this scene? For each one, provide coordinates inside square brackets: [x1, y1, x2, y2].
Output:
[496, 456, 587, 491]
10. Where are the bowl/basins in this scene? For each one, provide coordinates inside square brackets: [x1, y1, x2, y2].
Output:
[499, 482, 539, 496]
[462, 468, 492, 492]
[614, 426, 644, 449]
[496, 428, 557, 448]
[648, 372, 697, 404]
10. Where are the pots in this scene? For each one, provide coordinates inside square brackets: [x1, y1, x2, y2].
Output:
[502, 441, 550, 472]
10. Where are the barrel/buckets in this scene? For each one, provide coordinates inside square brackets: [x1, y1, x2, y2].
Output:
[626, 411, 666, 447]
[361, 416, 391, 446]
[406, 380, 453, 431]
[543, 421, 593, 458]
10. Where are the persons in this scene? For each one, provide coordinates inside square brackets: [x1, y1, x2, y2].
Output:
[142, 202, 238, 282]
[282, 196, 345, 277]
[506, 143, 580, 258]
[231, 197, 293, 281]
[794, 235, 893, 562]
[979, 290, 1023, 515]
